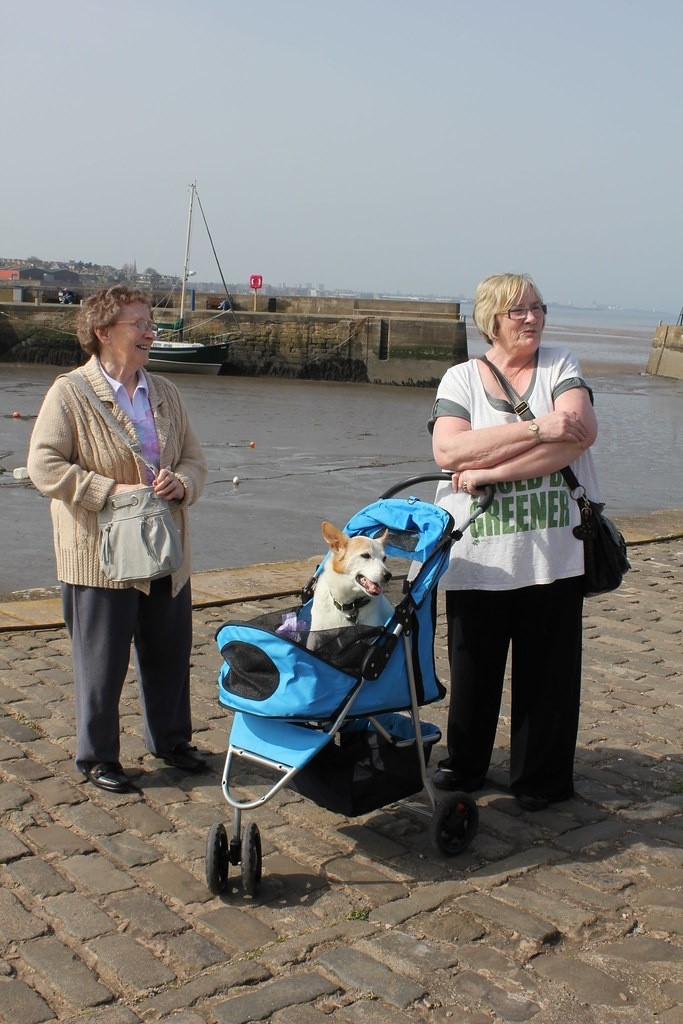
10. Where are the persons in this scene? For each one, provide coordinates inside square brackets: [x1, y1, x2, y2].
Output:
[62, 287, 74, 305]
[218, 294, 233, 311]
[26, 282, 209, 793]
[428, 271, 603, 812]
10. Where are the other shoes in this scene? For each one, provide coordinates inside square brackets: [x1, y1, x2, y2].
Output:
[519, 794, 548, 810]
[432, 768, 460, 790]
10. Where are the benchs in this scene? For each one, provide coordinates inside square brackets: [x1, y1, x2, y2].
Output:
[206, 296, 227, 309]
[42, 290, 80, 303]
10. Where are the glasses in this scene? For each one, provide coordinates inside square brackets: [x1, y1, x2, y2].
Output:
[500, 304, 547, 320]
[112, 317, 158, 334]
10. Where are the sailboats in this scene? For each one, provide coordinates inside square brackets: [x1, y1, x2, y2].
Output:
[150, 180, 241, 376]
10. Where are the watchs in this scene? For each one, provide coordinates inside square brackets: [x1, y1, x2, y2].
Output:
[529, 420, 540, 444]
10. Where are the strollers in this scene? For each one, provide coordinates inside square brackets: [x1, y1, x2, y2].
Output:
[203, 472, 494, 899]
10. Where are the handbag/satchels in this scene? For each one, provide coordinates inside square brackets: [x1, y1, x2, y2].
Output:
[96, 483, 185, 583]
[570, 487, 632, 597]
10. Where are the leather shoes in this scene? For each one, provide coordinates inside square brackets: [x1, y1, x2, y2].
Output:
[85, 761, 131, 793]
[151, 741, 206, 768]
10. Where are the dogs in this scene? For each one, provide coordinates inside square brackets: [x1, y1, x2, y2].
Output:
[306, 521, 395, 667]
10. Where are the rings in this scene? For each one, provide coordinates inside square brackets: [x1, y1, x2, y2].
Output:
[175, 481, 178, 485]
[462, 482, 468, 491]
